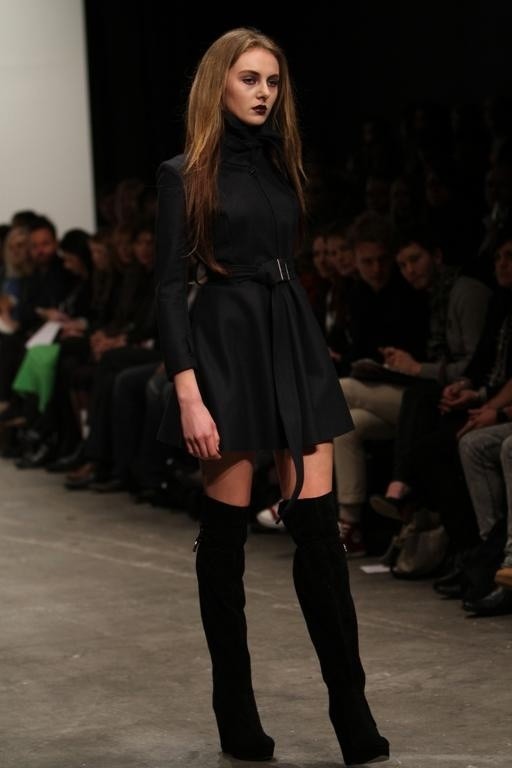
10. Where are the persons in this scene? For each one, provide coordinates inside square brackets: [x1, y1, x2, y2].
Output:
[257, 220, 511, 614]
[0, 209, 200, 521]
[142, 29, 391, 766]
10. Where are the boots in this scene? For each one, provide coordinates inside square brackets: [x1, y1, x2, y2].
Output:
[194, 496, 274, 763]
[278, 489, 390, 765]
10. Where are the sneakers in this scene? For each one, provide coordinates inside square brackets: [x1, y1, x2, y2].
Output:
[2, 423, 200, 515]
[432, 568, 467, 595]
[336, 518, 365, 558]
[257, 504, 285, 528]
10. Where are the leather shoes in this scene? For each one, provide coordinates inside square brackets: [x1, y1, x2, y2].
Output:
[462, 586, 511, 616]
[370, 493, 404, 520]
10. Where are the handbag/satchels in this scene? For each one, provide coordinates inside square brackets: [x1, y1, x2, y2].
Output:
[379, 525, 448, 580]
